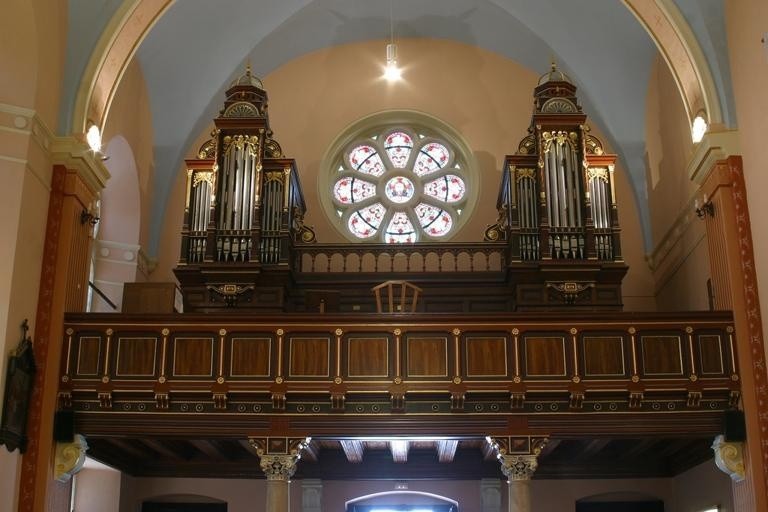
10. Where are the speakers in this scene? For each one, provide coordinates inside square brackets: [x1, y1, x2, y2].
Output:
[725, 411, 746, 443]
[57, 411, 74, 442]
